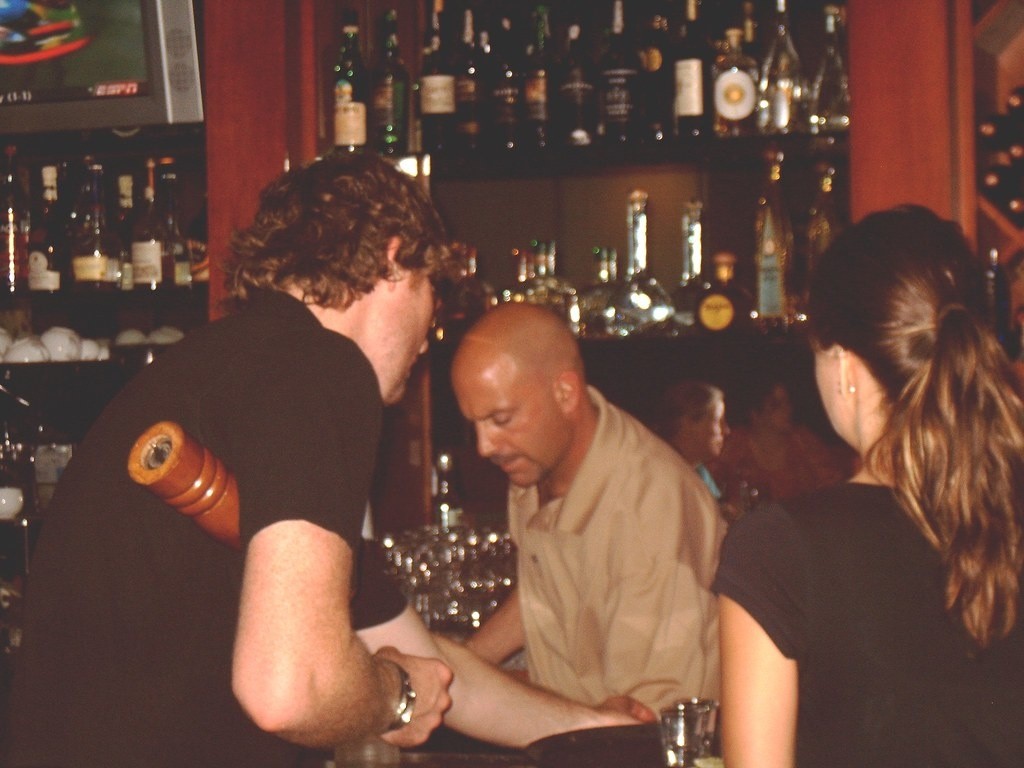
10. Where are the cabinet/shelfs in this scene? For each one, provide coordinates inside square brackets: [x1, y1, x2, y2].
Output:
[0, 0, 1022, 612]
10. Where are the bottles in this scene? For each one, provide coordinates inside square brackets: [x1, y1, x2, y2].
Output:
[977, 85, 1024, 353]
[754, 148, 799, 332]
[799, 161, 840, 321]
[670, 199, 706, 331]
[430, 452, 465, 529]
[434, 188, 671, 339]
[694, 250, 752, 332]
[810, 5, 850, 133]
[329, 1, 810, 156]
[0, 141, 194, 296]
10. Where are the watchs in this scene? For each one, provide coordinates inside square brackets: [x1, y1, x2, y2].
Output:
[378, 655, 416, 734]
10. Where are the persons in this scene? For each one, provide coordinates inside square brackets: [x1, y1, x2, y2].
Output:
[646, 380, 760, 521]
[461, 304, 729, 752]
[710, 203, 1024, 768]
[0, 155, 644, 768]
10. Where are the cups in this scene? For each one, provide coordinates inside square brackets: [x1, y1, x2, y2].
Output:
[0, 486, 25, 518]
[659, 697, 720, 767]
[334, 739, 401, 767]
[374, 525, 513, 632]
[0, 325, 112, 363]
